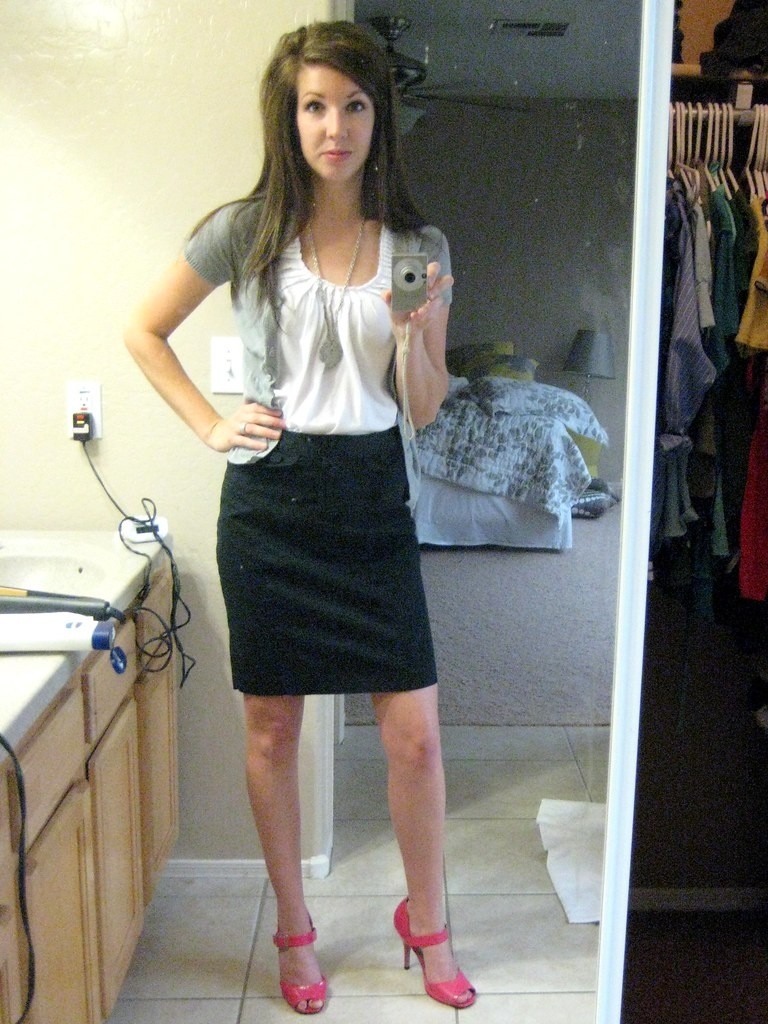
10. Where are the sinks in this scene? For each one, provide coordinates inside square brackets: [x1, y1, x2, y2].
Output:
[0, 514, 172, 763]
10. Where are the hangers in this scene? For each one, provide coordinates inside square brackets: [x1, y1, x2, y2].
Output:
[669, 101, 768, 236]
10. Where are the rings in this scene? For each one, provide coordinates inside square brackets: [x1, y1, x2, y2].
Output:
[240, 421, 249, 435]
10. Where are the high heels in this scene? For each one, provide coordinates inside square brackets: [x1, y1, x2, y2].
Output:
[393, 897, 476, 1008]
[273, 913, 327, 1014]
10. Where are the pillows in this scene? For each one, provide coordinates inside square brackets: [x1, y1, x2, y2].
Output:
[458, 375, 610, 448]
[571, 478, 619, 519]
[455, 355, 541, 381]
[445, 342, 515, 377]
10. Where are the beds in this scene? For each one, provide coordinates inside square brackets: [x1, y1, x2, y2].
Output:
[414, 374, 591, 551]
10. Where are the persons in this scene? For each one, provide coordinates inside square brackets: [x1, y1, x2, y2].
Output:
[122, 19, 478, 1021]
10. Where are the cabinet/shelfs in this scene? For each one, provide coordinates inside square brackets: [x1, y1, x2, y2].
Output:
[0, 570, 180, 1024]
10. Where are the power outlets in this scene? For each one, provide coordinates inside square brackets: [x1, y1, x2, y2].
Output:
[65, 383, 102, 440]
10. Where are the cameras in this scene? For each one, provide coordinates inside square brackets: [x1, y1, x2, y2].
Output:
[392, 253, 428, 312]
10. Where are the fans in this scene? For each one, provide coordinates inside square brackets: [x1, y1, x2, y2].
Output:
[368, 16, 533, 133]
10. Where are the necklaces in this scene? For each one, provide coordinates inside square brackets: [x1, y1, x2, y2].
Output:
[303, 215, 369, 372]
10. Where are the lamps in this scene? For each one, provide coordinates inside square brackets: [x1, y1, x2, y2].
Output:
[376, 41, 427, 131]
[560, 331, 615, 403]
